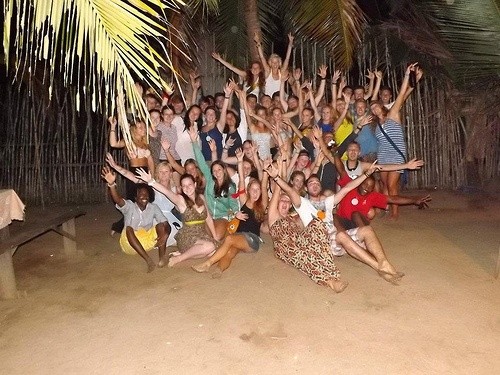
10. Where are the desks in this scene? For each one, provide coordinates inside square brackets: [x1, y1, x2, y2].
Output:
[0, 189, 26, 241]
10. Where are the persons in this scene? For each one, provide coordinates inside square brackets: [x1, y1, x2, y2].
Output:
[105, 30, 423, 223]
[329, 142, 431, 232]
[100, 165, 171, 272]
[189, 128, 249, 240]
[190, 148, 263, 278]
[267, 184, 349, 293]
[135, 167, 222, 268]
[312, 125, 424, 195]
[262, 160, 404, 285]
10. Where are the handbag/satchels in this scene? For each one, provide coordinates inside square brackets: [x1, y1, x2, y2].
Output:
[403, 169, 408, 184]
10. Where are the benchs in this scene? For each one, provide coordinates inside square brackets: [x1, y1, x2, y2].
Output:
[0, 209, 87, 298]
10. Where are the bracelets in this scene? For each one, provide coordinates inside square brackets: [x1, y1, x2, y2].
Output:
[357, 126, 361, 130]
[110, 130, 115, 132]
[321, 78, 325, 80]
[364, 172, 369, 178]
[225, 96, 229, 99]
[283, 160, 287, 162]
[108, 183, 115, 187]
[273, 175, 280, 182]
[332, 83, 336, 85]
[148, 180, 156, 185]
